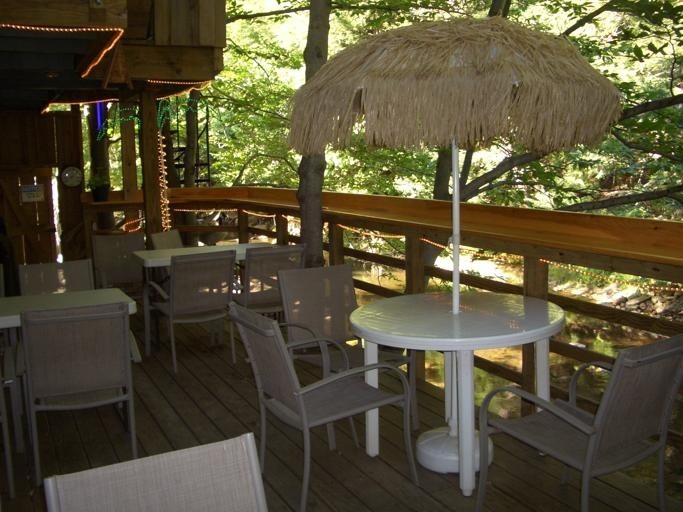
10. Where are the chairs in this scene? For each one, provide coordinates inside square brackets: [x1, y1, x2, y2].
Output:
[230, 242, 308, 362]
[94, 234, 146, 293]
[17, 258, 96, 296]
[226, 300, 420, 510]
[276, 264, 423, 452]
[475, 333, 681, 510]
[150, 232, 186, 279]
[43, 432, 268, 509]
[142, 249, 237, 374]
[23, 299, 138, 488]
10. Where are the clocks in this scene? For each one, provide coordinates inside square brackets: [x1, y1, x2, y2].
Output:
[60, 166, 83, 188]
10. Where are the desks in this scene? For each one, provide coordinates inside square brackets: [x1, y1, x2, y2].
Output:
[348, 289, 568, 476]
[134, 240, 281, 270]
[4, 285, 142, 452]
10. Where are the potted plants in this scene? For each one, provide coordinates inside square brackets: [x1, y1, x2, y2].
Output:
[81, 166, 123, 202]
